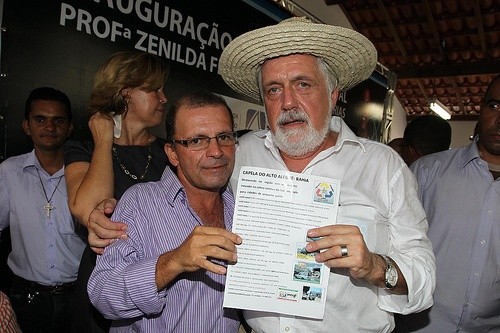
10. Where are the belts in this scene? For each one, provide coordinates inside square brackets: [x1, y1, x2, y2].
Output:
[16, 278, 74, 296]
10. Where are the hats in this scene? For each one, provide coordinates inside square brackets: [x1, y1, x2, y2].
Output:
[218, 16, 377, 101]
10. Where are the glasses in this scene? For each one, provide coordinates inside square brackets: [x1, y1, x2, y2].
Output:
[173, 131, 238, 151]
[399, 142, 416, 154]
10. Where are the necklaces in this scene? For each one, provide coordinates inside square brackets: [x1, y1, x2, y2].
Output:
[112, 139, 153, 180]
[35, 167, 64, 218]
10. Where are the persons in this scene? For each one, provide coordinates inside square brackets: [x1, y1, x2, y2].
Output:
[1, 88, 87, 328]
[63, 17, 500, 332]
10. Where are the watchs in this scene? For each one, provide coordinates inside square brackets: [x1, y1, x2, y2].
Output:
[380, 254, 398, 292]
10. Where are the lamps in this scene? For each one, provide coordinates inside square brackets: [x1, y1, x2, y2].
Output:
[430, 98, 452, 120]
